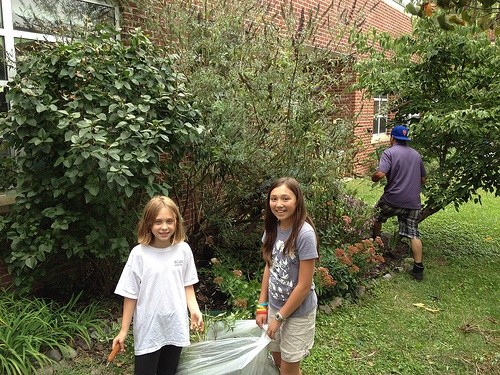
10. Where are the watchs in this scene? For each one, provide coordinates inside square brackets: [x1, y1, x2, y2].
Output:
[274, 312, 286, 322]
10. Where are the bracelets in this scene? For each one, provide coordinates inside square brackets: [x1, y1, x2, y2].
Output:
[256, 303, 268, 313]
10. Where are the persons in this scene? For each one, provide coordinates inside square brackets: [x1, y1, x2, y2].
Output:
[366, 126, 427, 280]
[256, 177, 317, 375]
[111, 195, 204, 375]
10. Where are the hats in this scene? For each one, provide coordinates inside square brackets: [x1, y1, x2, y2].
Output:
[391, 125, 409, 141]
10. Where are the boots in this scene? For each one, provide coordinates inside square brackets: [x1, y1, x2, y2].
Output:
[410, 263, 424, 280]
